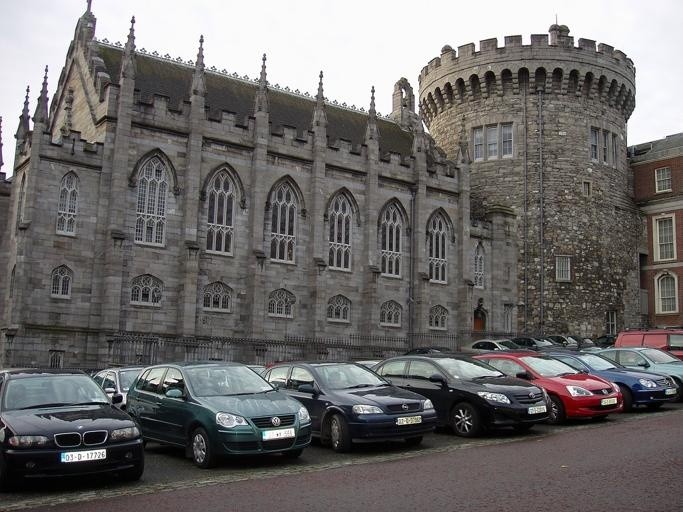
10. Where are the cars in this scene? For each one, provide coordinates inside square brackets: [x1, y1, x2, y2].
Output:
[259, 359, 442, 455]
[123, 358, 316, 470]
[0, 365, 149, 496]
[366, 351, 560, 443]
